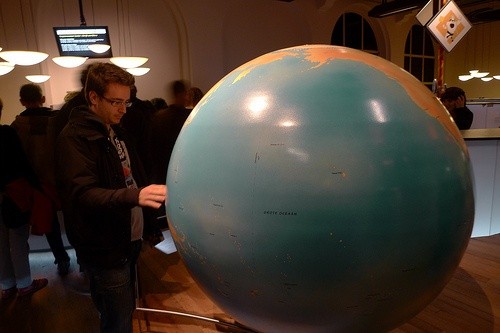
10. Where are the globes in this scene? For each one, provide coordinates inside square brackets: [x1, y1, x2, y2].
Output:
[164, 45, 478, 333]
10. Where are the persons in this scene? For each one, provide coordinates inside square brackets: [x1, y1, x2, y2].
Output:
[12, 83, 89, 277]
[0, 101, 49, 297]
[113, 80, 203, 248]
[441, 86, 473, 130]
[56, 64, 90, 135]
[56, 62, 166, 333]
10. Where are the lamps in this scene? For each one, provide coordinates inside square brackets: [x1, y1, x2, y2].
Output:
[0, 0, 152, 84]
[458, 9, 500, 81]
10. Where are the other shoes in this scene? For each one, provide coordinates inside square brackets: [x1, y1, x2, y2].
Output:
[57, 263, 70, 272]
[18, 279, 49, 297]
[1, 284, 18, 299]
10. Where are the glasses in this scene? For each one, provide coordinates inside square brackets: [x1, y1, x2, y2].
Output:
[101, 96, 133, 108]
